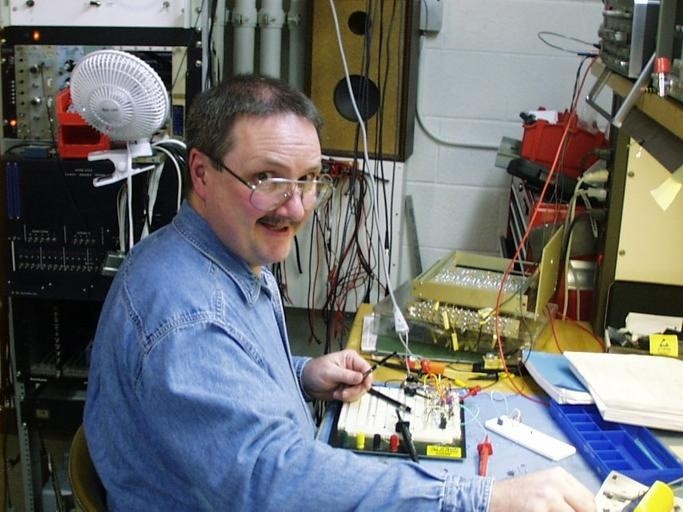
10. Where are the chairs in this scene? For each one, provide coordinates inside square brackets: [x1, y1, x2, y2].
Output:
[65, 420, 106, 512]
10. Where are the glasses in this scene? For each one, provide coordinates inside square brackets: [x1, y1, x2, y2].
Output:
[215, 159, 334, 215]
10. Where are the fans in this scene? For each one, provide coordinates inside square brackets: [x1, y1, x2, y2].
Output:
[68, 48, 171, 188]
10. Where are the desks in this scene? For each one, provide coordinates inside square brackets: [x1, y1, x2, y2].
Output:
[314, 302, 682, 512]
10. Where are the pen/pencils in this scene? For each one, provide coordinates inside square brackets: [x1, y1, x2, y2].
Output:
[363, 350, 397, 377]
[367, 388, 412, 412]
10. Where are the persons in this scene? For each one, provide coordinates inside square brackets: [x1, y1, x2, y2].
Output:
[81, 70, 601, 511]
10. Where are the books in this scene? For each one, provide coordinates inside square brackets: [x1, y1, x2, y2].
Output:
[561, 349, 683, 434]
[518, 348, 593, 407]
